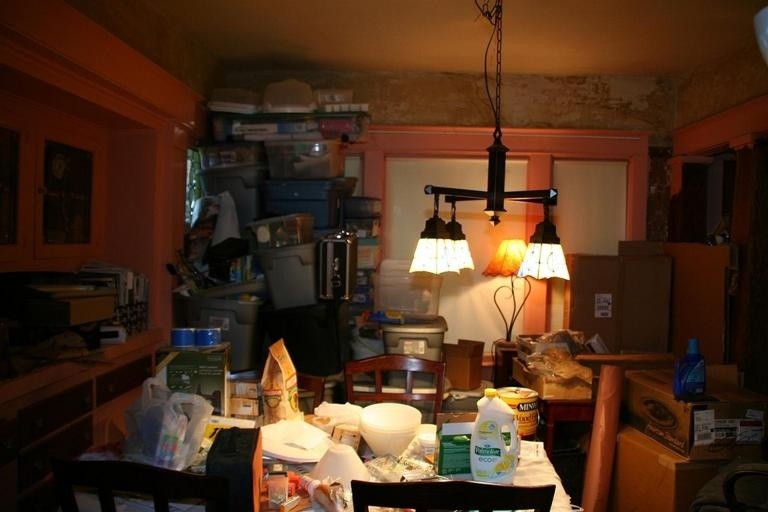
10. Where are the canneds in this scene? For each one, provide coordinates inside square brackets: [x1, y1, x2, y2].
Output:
[170, 325, 194, 347]
[194, 326, 222, 348]
[498, 387, 540, 438]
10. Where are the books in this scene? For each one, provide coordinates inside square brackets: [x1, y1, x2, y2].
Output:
[78, 267, 149, 335]
[211, 116, 360, 141]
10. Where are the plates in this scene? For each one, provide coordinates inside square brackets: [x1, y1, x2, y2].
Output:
[254, 422, 337, 464]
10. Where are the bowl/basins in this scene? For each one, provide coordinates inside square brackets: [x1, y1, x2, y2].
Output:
[358, 401, 423, 457]
[316, 445, 369, 494]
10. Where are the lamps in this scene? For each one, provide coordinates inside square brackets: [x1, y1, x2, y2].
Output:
[407, 2, 571, 281]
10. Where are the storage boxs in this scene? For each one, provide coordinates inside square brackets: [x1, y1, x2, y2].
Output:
[608, 371, 767, 511]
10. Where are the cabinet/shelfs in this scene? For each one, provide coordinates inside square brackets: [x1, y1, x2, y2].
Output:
[0, 90, 111, 273]
[564, 251, 672, 353]
[1, 329, 160, 512]
